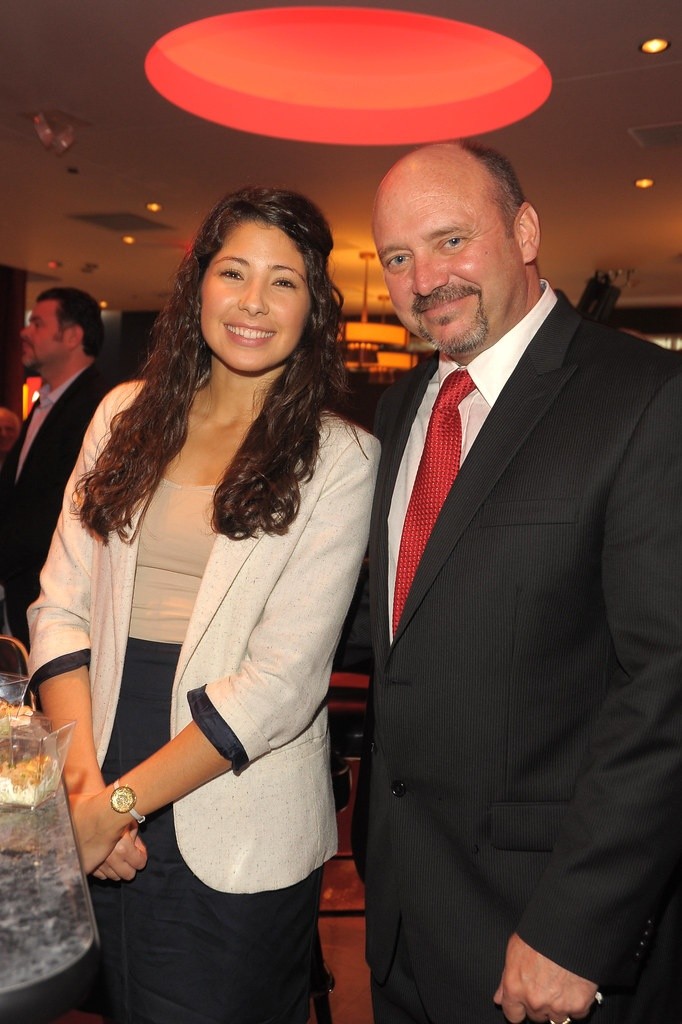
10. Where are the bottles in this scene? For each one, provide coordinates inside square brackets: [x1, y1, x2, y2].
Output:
[0, 674, 95, 994]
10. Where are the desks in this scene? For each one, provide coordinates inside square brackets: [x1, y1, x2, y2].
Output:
[0, 711, 100, 1024]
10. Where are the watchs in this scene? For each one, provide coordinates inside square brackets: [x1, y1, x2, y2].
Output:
[110, 779, 145, 824]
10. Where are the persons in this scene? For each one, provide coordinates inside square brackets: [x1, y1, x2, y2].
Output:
[346, 142, 682, 1024]
[0, 287, 123, 653]
[26, 186, 384, 1024]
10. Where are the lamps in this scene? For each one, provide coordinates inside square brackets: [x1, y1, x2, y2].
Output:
[337, 252, 419, 384]
[22, 110, 97, 156]
[143, 0, 553, 148]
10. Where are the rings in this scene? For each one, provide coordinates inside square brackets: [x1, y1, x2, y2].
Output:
[550, 1017, 570, 1024]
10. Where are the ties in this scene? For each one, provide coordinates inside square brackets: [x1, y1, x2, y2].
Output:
[393, 369, 477, 637]
